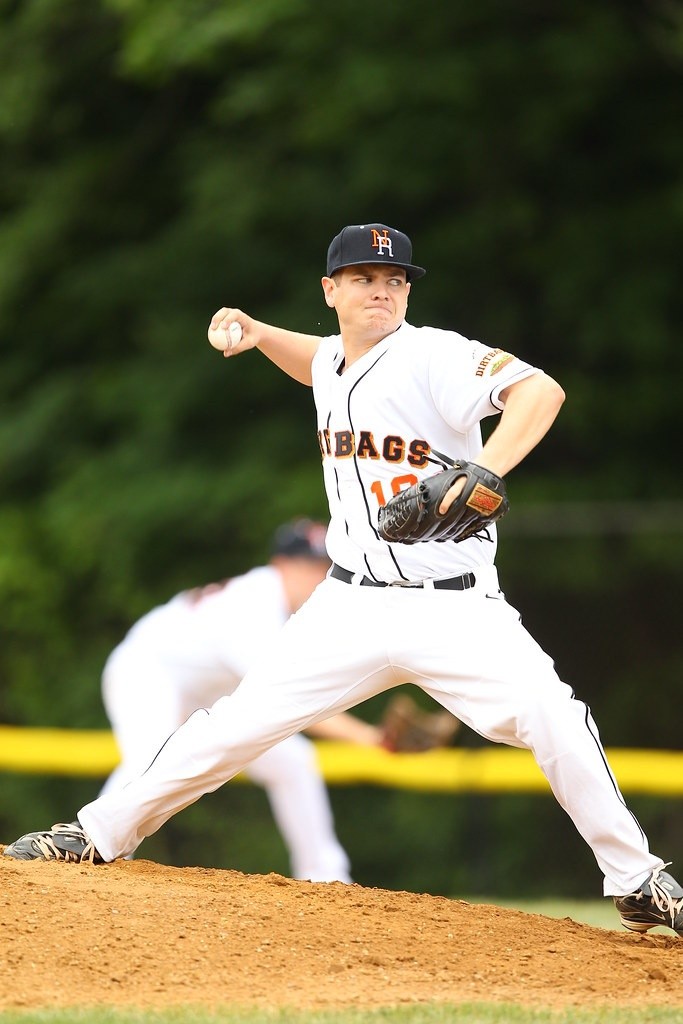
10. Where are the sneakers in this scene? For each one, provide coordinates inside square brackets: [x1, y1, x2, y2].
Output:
[612, 862, 683, 937]
[4, 820, 117, 864]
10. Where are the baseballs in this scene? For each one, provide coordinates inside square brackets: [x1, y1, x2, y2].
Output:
[207, 318, 243, 352]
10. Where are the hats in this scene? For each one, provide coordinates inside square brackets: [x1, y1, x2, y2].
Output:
[327, 223, 426, 278]
[265, 516, 330, 563]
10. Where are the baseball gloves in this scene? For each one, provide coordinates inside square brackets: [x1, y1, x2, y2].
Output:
[373, 461, 510, 545]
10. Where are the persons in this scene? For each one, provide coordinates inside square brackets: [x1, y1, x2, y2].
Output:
[97, 516, 456, 884]
[0, 224, 683, 939]
